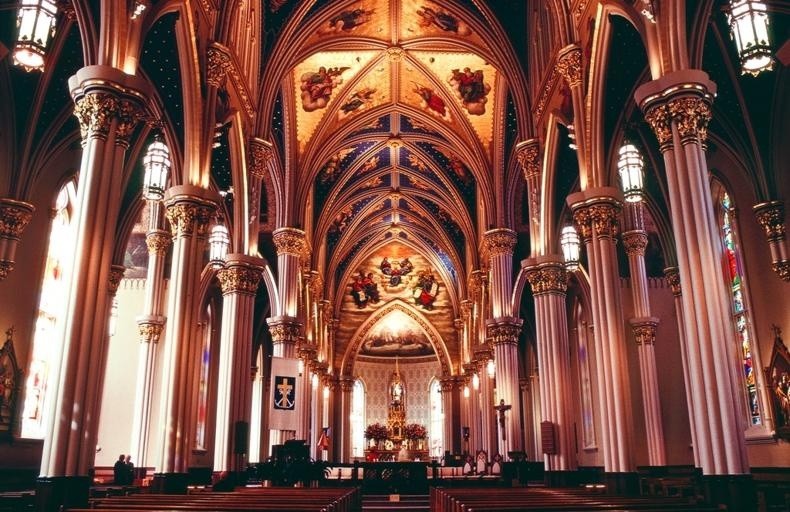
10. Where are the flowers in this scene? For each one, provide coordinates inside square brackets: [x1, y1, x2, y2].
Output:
[402, 425, 428, 438]
[363, 423, 390, 438]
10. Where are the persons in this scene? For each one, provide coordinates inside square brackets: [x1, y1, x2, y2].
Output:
[114, 454, 125, 484]
[458, 67, 475, 101]
[124, 455, 133, 489]
[352, 258, 439, 310]
[307, 67, 332, 101]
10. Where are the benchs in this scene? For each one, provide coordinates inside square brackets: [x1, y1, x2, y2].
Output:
[430, 486, 723, 512]
[60, 487, 362, 511]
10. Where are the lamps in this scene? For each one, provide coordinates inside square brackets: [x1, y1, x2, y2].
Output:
[472, 373, 480, 391]
[207, 224, 231, 270]
[616, 135, 647, 204]
[12, 0, 58, 72]
[722, 0, 775, 78]
[312, 372, 319, 388]
[462, 383, 471, 399]
[298, 357, 304, 376]
[560, 225, 582, 273]
[142, 133, 172, 202]
[323, 386, 329, 401]
[486, 358, 495, 380]
